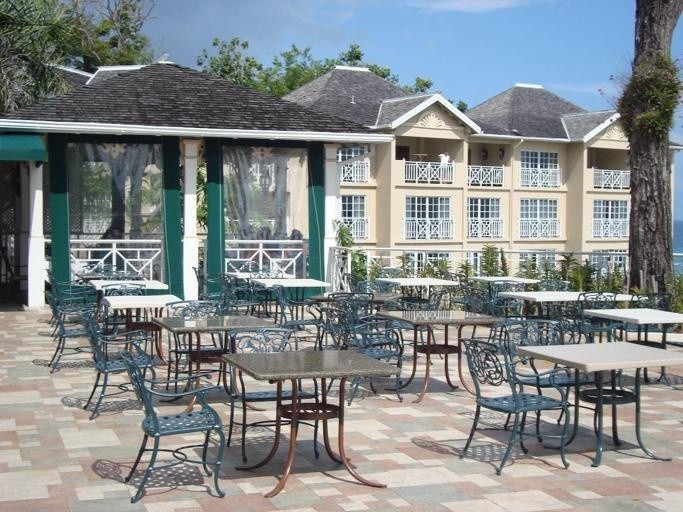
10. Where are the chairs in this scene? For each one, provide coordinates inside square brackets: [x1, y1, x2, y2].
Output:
[1, 247, 28, 301]
